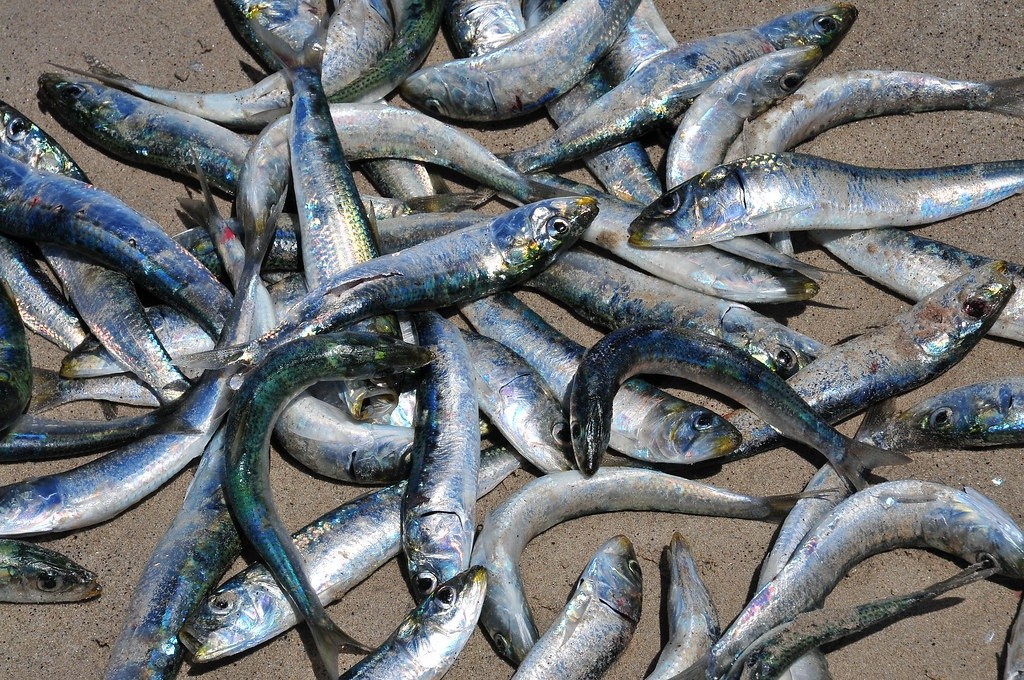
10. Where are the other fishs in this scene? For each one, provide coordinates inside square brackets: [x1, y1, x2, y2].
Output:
[0, 0, 1024, 680]
